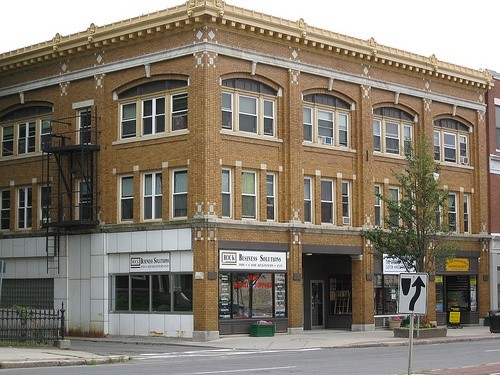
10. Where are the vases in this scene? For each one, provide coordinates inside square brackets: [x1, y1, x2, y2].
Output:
[389, 321, 401, 330]
[250, 324, 274, 337]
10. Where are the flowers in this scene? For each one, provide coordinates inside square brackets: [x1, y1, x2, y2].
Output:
[256, 320, 272, 324]
[388, 317, 402, 321]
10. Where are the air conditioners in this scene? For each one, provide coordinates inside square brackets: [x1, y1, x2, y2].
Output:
[461, 156, 469, 164]
[322, 137, 332, 145]
[342, 217, 350, 224]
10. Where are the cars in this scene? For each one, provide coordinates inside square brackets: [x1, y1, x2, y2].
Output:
[228, 303, 253, 315]
